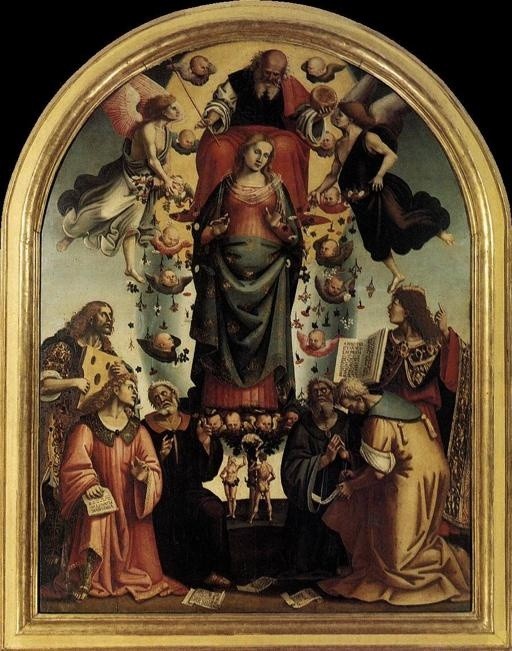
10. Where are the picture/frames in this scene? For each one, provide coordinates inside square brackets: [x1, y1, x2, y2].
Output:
[1, 0, 512, 651]
